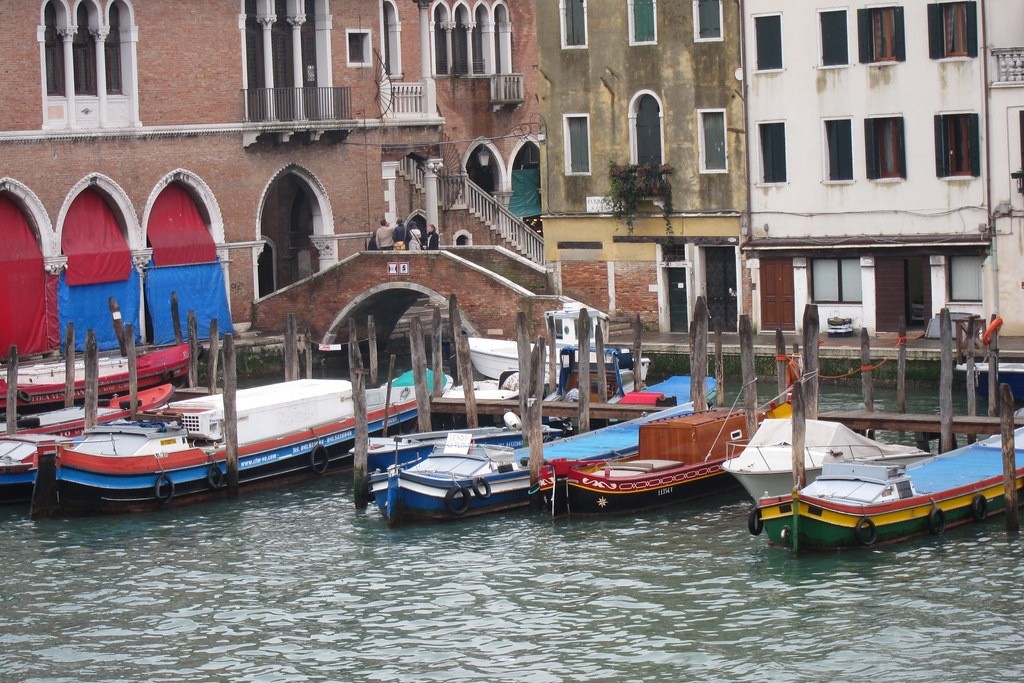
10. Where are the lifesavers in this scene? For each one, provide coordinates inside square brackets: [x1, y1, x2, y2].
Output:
[154, 473, 174, 503]
[472, 476, 491, 498]
[207, 465, 223, 490]
[928, 508, 947, 535]
[17, 417, 41, 428]
[400, 387, 411, 397]
[311, 444, 329, 475]
[162, 369, 174, 384]
[747, 507, 764, 536]
[971, 494, 988, 522]
[444, 486, 472, 515]
[0, 413, 21, 421]
[856, 517, 877, 544]
[18, 389, 32, 403]
[981, 318, 1003, 345]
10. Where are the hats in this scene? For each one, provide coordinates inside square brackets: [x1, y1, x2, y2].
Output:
[408, 221, 416, 228]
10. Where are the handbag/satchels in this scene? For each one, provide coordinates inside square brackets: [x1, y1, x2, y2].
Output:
[420, 243, 424, 250]
[394, 241, 405, 250]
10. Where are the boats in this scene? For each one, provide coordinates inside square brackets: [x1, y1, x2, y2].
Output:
[348, 398, 792, 528]
[721, 419, 935, 503]
[0, 344, 453, 518]
[749, 427, 1024, 550]
[468, 337, 651, 381]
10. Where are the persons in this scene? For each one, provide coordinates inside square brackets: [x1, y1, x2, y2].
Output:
[368, 231, 376, 250]
[405, 221, 421, 250]
[424, 224, 438, 250]
[393, 219, 412, 249]
[376, 219, 395, 249]
